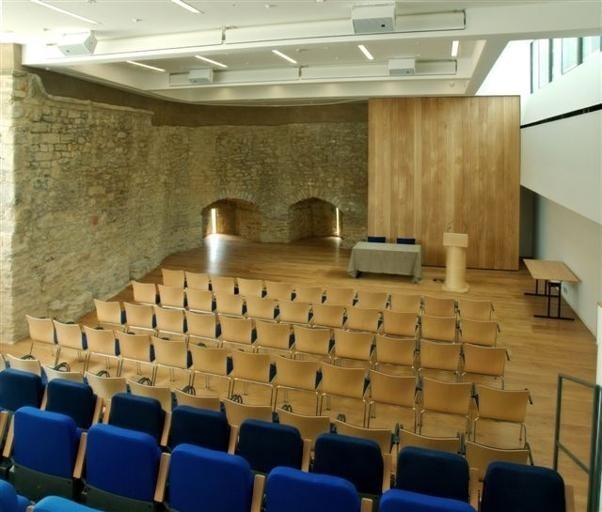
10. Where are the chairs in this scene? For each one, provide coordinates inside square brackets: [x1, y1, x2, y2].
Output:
[397, 239, 415, 244]
[368, 237, 385, 242]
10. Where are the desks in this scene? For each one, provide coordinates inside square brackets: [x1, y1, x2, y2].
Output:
[347, 242, 421, 283]
[523, 259, 578, 321]
